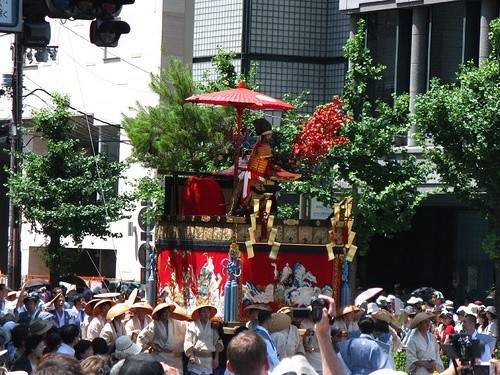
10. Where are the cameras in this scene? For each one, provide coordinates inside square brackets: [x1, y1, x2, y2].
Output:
[292, 295, 326, 321]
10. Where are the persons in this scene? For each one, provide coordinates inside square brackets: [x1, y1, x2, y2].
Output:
[231, 118, 273, 217]
[0, 278, 500, 375]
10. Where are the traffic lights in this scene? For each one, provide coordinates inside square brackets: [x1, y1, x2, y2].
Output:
[91, 1, 135, 48]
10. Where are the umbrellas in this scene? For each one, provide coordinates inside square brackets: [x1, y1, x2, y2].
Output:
[59, 272, 89, 288]
[353, 288, 383, 307]
[210, 160, 303, 181]
[185, 83, 298, 194]
[19, 278, 50, 290]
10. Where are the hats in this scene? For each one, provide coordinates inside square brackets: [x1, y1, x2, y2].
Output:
[115, 335, 142, 359]
[277, 307, 292, 314]
[366, 302, 379, 314]
[268, 313, 292, 333]
[336, 304, 360, 316]
[484, 306, 497, 315]
[109, 353, 165, 375]
[243, 304, 270, 318]
[386, 295, 396, 302]
[191, 305, 217, 321]
[27, 317, 53, 336]
[376, 294, 387, 306]
[444, 300, 454, 308]
[409, 312, 436, 328]
[85, 288, 186, 320]
